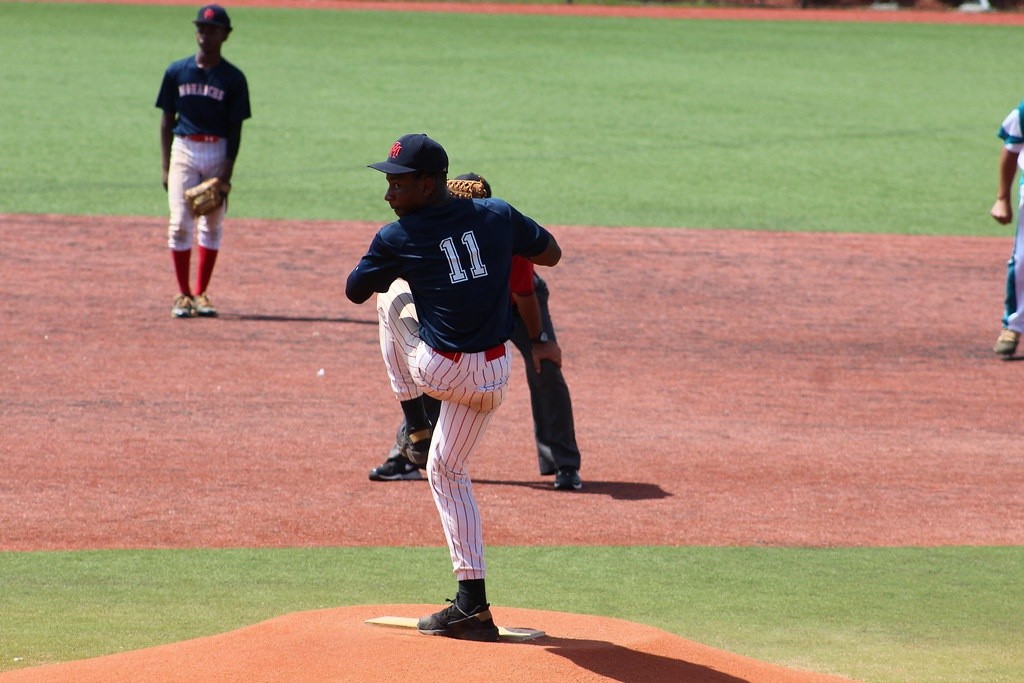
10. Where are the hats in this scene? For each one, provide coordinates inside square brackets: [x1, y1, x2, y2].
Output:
[453, 172, 491, 198]
[367, 133, 449, 174]
[193, 4, 232, 31]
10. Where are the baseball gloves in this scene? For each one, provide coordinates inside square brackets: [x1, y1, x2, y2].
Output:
[185, 176, 230, 217]
[447, 179, 486, 199]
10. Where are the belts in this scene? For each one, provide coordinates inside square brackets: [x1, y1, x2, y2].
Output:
[178, 134, 220, 143]
[433, 344, 505, 363]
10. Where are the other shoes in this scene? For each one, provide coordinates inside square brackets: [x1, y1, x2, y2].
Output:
[993, 328, 1020, 354]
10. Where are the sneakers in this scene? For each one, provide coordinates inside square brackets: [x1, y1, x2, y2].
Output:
[417, 593, 499, 641]
[193, 292, 217, 315]
[554, 467, 582, 489]
[170, 293, 194, 318]
[396, 419, 432, 464]
[369, 455, 422, 480]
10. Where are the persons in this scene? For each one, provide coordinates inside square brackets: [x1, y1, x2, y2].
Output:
[990, 100, 1024, 356]
[153, 3, 252, 318]
[369, 172, 582, 491]
[345, 133, 562, 642]
